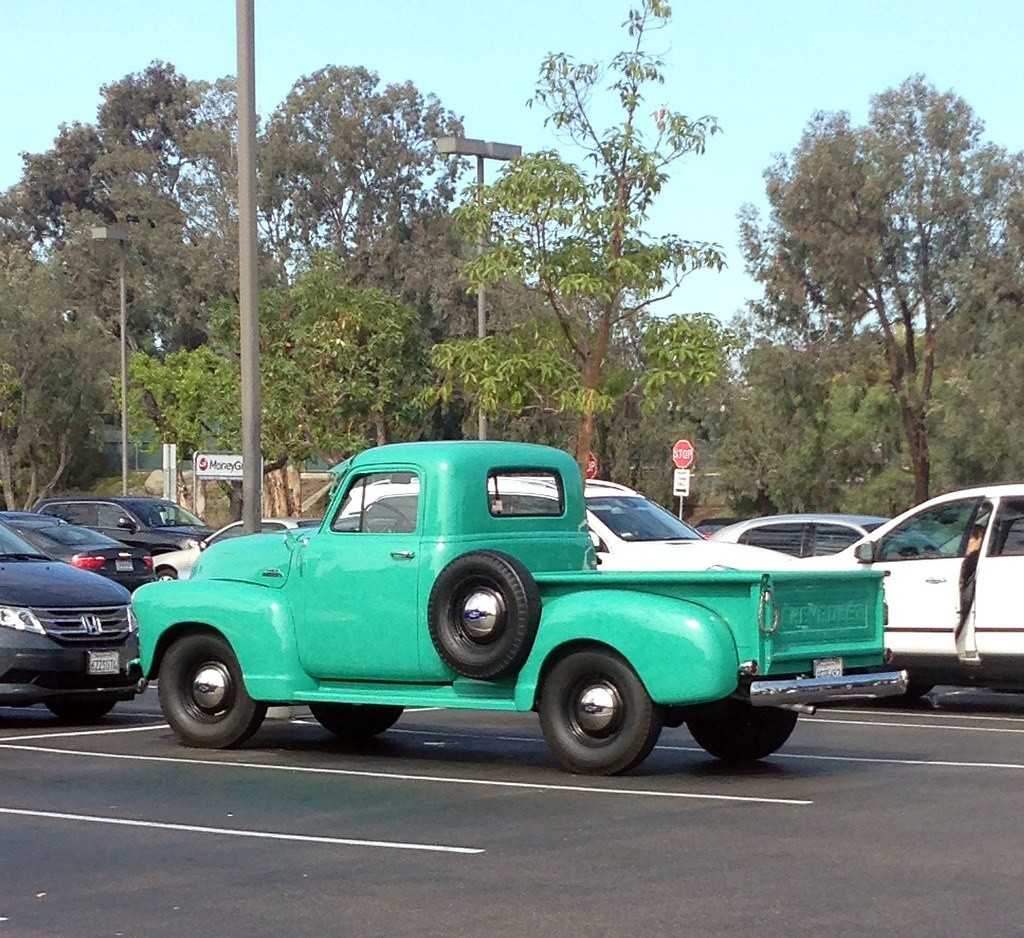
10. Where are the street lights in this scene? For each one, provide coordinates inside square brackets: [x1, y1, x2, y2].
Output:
[89, 225, 130, 498]
[434, 135, 521, 443]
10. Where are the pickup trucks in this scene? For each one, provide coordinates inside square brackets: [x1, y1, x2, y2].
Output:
[128, 436, 912, 777]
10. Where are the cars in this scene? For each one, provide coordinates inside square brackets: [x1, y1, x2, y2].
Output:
[0, 517, 143, 725]
[802, 480, 1024, 706]
[708, 512, 947, 564]
[0, 510, 161, 596]
[149, 514, 322, 583]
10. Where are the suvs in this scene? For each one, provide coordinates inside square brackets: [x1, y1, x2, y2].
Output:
[336, 474, 801, 573]
[29, 495, 223, 559]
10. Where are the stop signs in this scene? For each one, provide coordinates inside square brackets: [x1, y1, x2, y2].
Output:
[585, 452, 597, 479]
[672, 439, 694, 469]
